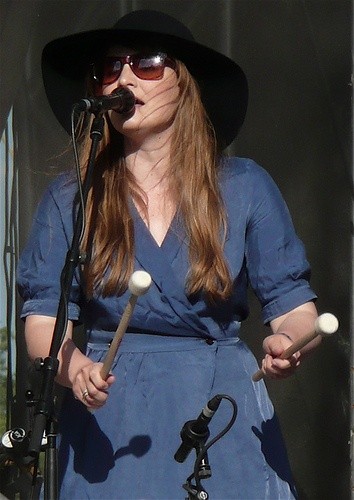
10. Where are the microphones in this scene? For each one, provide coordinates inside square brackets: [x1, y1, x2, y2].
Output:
[77, 85, 135, 113]
[174, 394, 222, 463]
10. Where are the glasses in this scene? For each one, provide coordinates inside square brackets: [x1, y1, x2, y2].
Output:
[87, 50, 179, 85]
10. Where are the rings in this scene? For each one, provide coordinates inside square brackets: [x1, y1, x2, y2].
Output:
[83, 389, 88, 398]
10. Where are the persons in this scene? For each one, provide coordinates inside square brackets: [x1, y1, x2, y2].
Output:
[16, 11, 318, 500]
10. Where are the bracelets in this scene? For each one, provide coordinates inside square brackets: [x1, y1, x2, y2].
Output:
[280, 333, 294, 344]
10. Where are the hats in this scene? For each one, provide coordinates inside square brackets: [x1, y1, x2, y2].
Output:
[41, 9, 249, 151]
